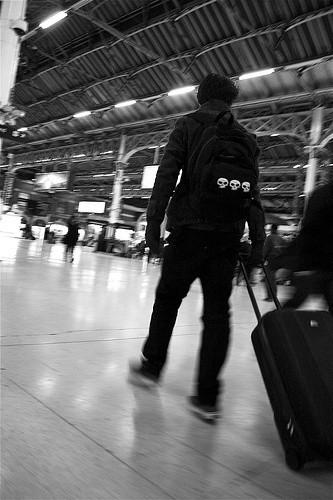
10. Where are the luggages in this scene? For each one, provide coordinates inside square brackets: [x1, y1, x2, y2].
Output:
[239, 252, 333, 471]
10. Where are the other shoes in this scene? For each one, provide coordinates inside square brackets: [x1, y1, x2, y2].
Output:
[186, 395, 221, 419]
[132, 366, 158, 387]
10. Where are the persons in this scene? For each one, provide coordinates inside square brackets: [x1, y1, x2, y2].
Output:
[261, 224, 288, 301]
[271, 173, 333, 314]
[128, 74, 266, 420]
[234, 236, 257, 286]
[62, 216, 80, 263]
[134, 225, 146, 260]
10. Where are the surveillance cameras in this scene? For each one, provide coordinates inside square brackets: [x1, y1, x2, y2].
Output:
[13, 20, 29, 35]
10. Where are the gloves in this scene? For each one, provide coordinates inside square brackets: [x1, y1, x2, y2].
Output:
[145, 222, 161, 251]
[245, 241, 263, 266]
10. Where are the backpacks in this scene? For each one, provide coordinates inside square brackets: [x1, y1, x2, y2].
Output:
[181, 111, 261, 224]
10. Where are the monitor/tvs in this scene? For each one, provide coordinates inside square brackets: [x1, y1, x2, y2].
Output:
[33, 171, 70, 191]
[140, 164, 182, 189]
